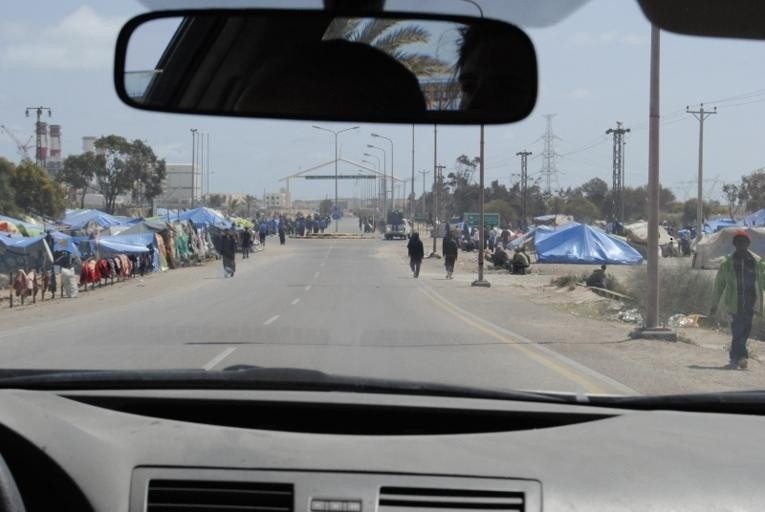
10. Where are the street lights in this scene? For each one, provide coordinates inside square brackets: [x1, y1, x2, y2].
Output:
[312, 125, 359, 235]
[359, 132, 394, 222]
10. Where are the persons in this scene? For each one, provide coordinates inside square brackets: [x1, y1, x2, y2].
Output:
[449, 26, 518, 112]
[232, 38, 430, 113]
[210, 209, 333, 279]
[709, 228, 764, 369]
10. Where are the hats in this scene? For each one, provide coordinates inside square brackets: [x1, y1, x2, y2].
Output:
[733, 230, 750, 244]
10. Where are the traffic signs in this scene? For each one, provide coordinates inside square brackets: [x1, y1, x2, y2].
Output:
[465, 212, 500, 226]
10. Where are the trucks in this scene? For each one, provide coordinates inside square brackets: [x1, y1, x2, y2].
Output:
[385, 219, 410, 240]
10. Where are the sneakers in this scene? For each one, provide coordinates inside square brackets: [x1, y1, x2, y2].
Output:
[731, 359, 748, 369]
